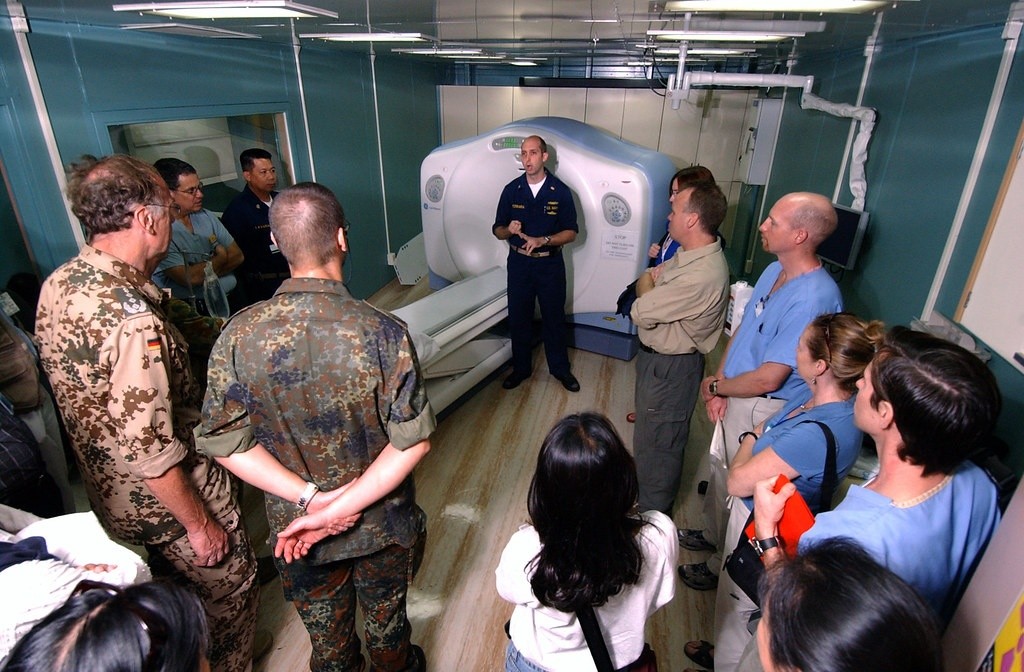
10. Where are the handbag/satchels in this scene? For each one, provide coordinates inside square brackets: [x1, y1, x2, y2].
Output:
[615, 232, 671, 316]
[628, 642, 658, 672]
[722, 420, 838, 610]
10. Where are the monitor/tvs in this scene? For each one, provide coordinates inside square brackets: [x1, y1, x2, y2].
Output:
[813, 203, 870, 271]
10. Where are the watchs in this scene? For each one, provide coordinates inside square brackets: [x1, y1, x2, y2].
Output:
[544, 236, 551, 245]
[710, 379, 728, 398]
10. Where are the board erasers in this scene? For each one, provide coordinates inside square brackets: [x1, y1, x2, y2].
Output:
[1012, 352, 1024, 366]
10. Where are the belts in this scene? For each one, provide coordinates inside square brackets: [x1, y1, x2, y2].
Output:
[640, 342, 658, 354]
[511, 245, 550, 258]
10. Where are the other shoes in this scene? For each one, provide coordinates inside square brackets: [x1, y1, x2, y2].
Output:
[256, 555, 281, 586]
[626, 413, 636, 422]
[252, 629, 273, 662]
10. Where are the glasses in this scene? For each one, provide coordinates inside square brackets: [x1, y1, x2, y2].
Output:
[143, 203, 181, 217]
[169, 182, 204, 194]
[341, 220, 351, 231]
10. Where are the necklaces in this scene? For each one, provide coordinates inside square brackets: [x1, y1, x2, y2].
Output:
[801, 399, 812, 409]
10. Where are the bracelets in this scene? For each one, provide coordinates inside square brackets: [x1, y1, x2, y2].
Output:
[739, 432, 757, 444]
[298, 481, 319, 512]
[751, 536, 784, 556]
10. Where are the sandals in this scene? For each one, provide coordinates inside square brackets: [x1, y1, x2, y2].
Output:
[684, 640, 715, 670]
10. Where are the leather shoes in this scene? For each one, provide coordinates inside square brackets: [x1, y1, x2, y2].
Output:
[503, 371, 530, 388]
[554, 372, 580, 391]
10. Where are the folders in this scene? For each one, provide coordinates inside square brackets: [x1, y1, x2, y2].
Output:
[744, 473, 816, 565]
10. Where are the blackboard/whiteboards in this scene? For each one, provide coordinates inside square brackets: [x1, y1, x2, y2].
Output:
[954, 121, 1024, 375]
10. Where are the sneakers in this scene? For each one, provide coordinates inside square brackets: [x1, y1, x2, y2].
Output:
[678, 561, 719, 590]
[676, 529, 716, 550]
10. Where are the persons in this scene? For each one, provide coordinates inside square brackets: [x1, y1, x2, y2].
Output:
[220, 149, 291, 310]
[152, 159, 243, 411]
[685, 313, 886, 672]
[494, 412, 680, 672]
[615, 165, 728, 519]
[33, 155, 258, 672]
[677, 192, 843, 589]
[493, 136, 580, 392]
[735, 325, 1000, 672]
[754, 536, 941, 672]
[0, 307, 75, 518]
[195, 182, 437, 672]
[0, 498, 212, 672]
